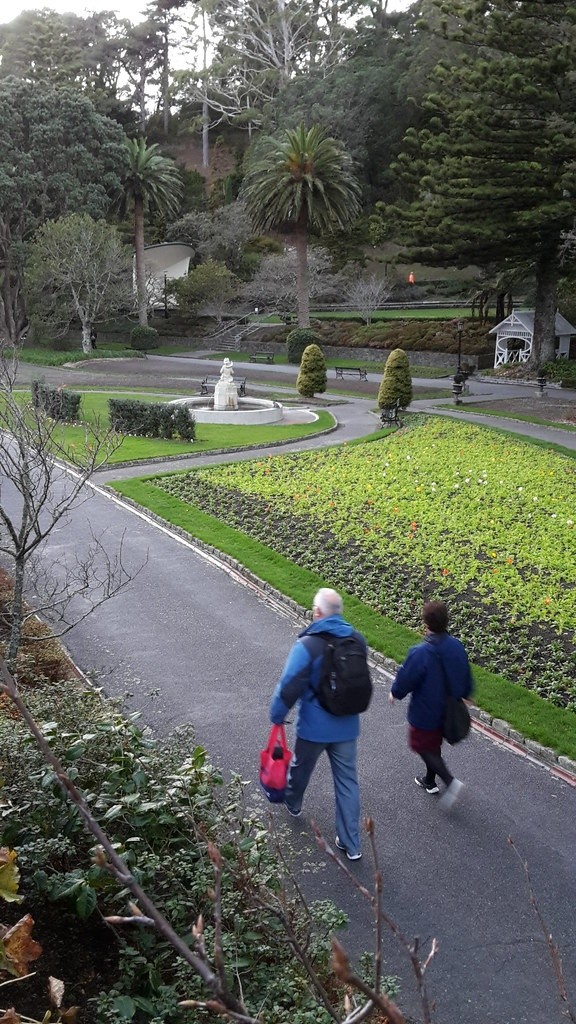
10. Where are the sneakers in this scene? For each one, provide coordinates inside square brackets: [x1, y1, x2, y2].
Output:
[283, 802, 303, 817]
[335, 835, 362, 859]
[415, 776, 439, 794]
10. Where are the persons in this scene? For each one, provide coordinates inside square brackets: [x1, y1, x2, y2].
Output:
[268, 587, 373, 860]
[409, 271, 415, 284]
[388, 600, 474, 812]
[219, 358, 234, 384]
[91, 327, 98, 349]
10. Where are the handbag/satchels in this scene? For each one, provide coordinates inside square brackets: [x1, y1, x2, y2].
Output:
[259, 724, 292, 804]
[443, 694, 471, 744]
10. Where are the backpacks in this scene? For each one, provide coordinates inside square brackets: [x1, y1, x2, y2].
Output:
[308, 631, 372, 716]
